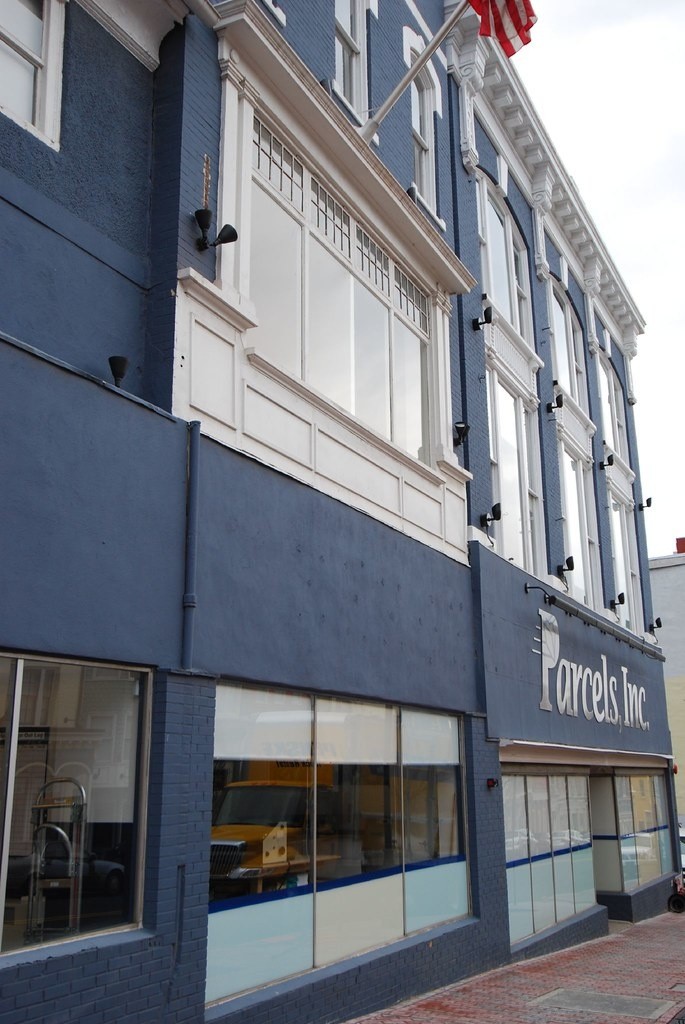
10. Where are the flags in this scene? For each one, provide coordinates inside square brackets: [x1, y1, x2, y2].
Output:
[469, 0, 538, 59]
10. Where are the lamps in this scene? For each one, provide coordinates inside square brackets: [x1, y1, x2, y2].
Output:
[610, 592, 624, 609]
[547, 394, 563, 413]
[407, 186, 417, 203]
[649, 617, 662, 632]
[600, 454, 613, 470]
[480, 503, 501, 527]
[557, 556, 574, 576]
[473, 306, 492, 330]
[319, 79, 331, 95]
[639, 497, 651, 511]
[108, 356, 130, 388]
[453, 422, 471, 448]
[195, 209, 239, 252]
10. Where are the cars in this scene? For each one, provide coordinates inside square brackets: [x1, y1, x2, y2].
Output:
[678, 827, 685, 887]
[209, 779, 339, 894]
[546, 829, 585, 851]
[621, 831, 657, 870]
[9, 844, 125, 896]
[505, 828, 536, 854]
